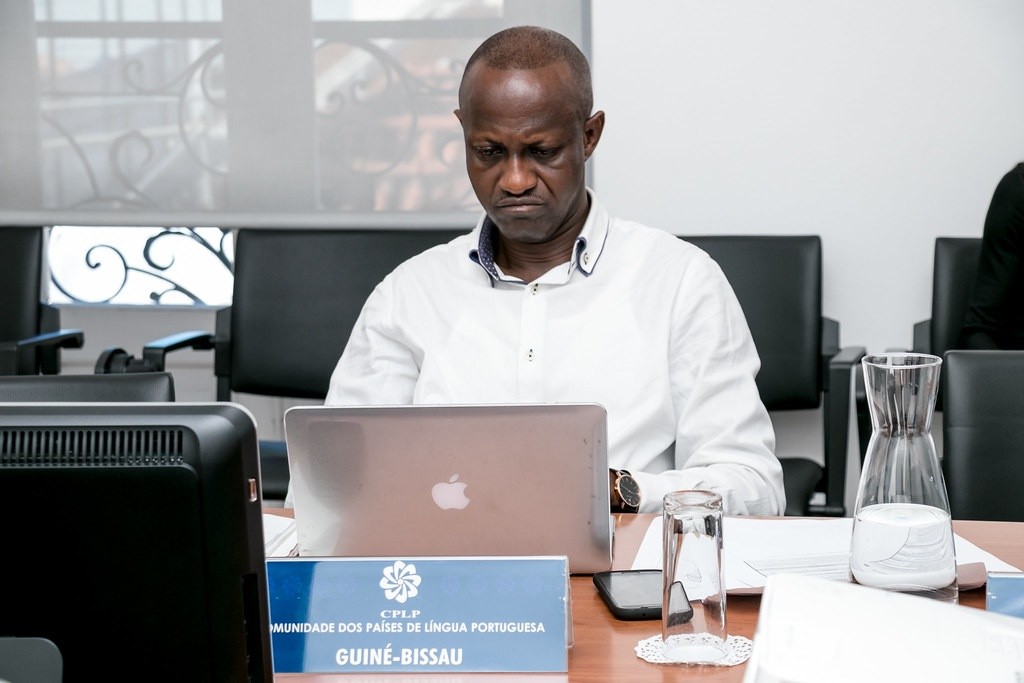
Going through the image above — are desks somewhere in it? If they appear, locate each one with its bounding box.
[262,500,1024,682]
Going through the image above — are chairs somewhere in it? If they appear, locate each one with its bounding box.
[675,233,868,517]
[93,204,473,508]
[906,164,1024,522]
[0,197,85,376]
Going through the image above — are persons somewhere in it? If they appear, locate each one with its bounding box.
[284,27,786,519]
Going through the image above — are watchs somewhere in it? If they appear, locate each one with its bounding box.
[609,466,642,515]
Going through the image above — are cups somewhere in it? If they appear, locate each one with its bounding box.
[661,490,727,646]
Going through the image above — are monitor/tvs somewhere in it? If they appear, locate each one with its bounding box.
[0,400,275,682]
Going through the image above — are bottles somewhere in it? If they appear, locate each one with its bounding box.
[850,350,960,606]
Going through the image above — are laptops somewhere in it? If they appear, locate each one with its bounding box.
[282,401,615,575]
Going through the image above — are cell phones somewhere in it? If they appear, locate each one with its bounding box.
[593,570,692,623]
